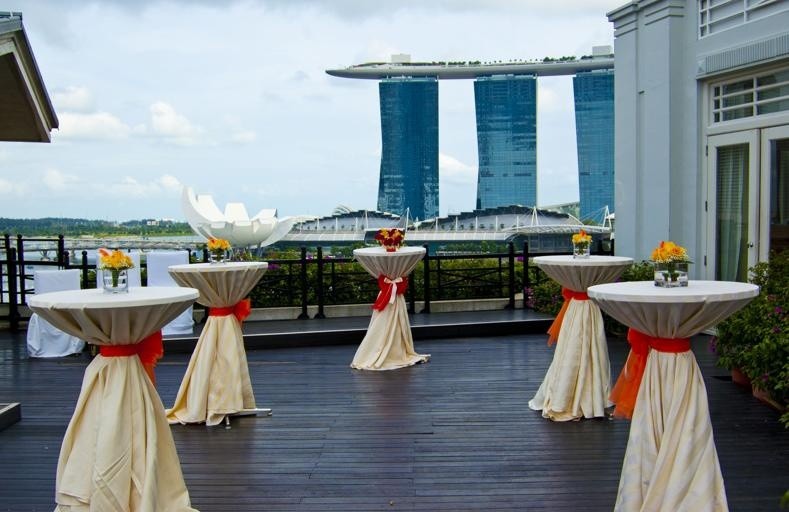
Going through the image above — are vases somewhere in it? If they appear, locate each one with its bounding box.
[653,266,688,287]
[101,267,128,293]
[573,243,590,256]
[208,248,231,262]
[386,243,397,252]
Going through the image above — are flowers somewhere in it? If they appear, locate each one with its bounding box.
[96,246,136,289]
[572,230,593,255]
[374,229,405,252]
[649,241,695,287]
[712,250,789,412]
[206,239,232,262]
